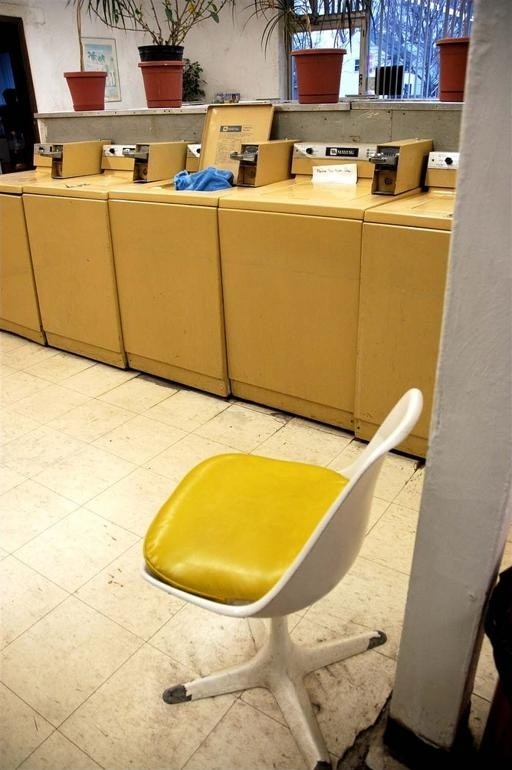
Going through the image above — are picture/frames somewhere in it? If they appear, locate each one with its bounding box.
[80,37,121,102]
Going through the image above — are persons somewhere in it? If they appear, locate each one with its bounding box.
[2,86,19,104]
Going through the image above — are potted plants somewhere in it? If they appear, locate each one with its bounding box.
[88,0,236,108]
[63,0,107,111]
[240,0,376,104]
[435,0,472,102]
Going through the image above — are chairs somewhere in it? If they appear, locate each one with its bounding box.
[142,387,423,769]
